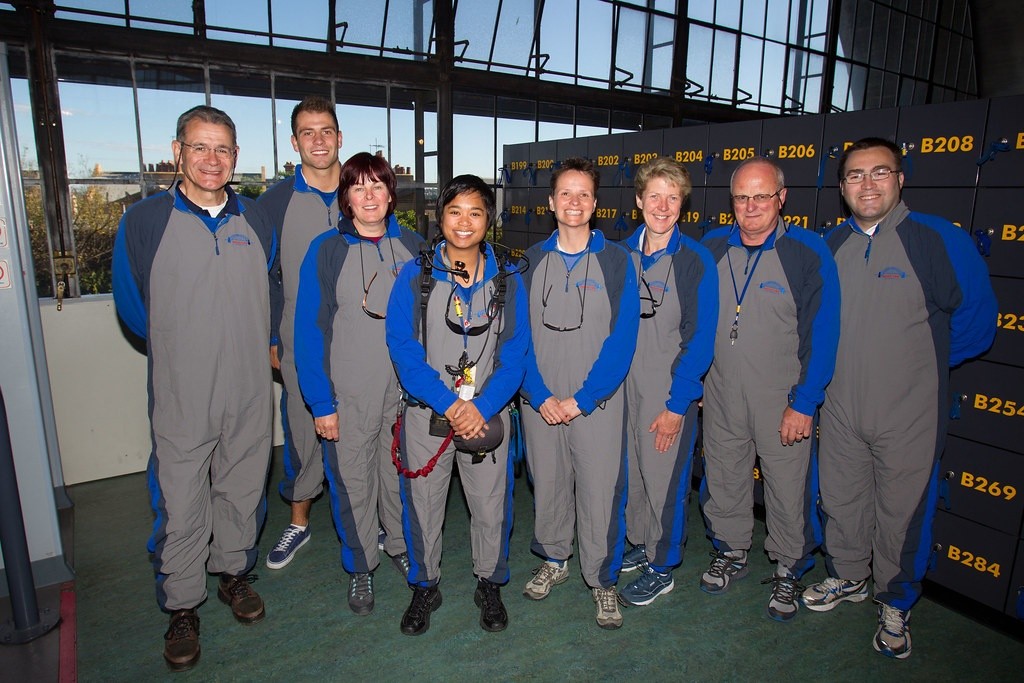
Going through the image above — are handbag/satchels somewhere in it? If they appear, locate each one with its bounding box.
[450,413,505,457]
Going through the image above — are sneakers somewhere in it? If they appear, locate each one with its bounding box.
[347,572,374,615]
[400,587,442,636]
[872,603,911,659]
[393,550,415,589]
[620,544,649,572]
[217,572,264,625]
[619,565,674,607]
[592,586,628,631]
[759,559,808,621]
[162,612,201,671]
[266,524,310,569]
[698,549,749,595]
[521,559,569,601]
[375,521,387,553]
[473,576,508,631]
[801,576,869,611]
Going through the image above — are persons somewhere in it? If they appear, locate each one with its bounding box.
[385,173,532,635]
[515,153,640,630]
[112,105,286,671]
[700,156,843,622]
[256,94,345,568]
[294,152,430,614]
[616,157,719,606]
[802,137,1000,660]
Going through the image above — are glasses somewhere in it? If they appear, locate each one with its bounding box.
[444,287,493,337]
[730,188,783,204]
[840,169,902,184]
[639,275,657,319]
[361,274,387,320]
[180,141,238,159]
[541,284,583,333]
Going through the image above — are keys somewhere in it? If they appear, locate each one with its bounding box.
[730,330,737,346]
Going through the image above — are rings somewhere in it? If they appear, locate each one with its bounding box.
[796,432,803,434]
[667,436,672,439]
[550,419,554,421]
[321,431,325,434]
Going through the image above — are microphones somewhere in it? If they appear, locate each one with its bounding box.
[426,255,468,275]
